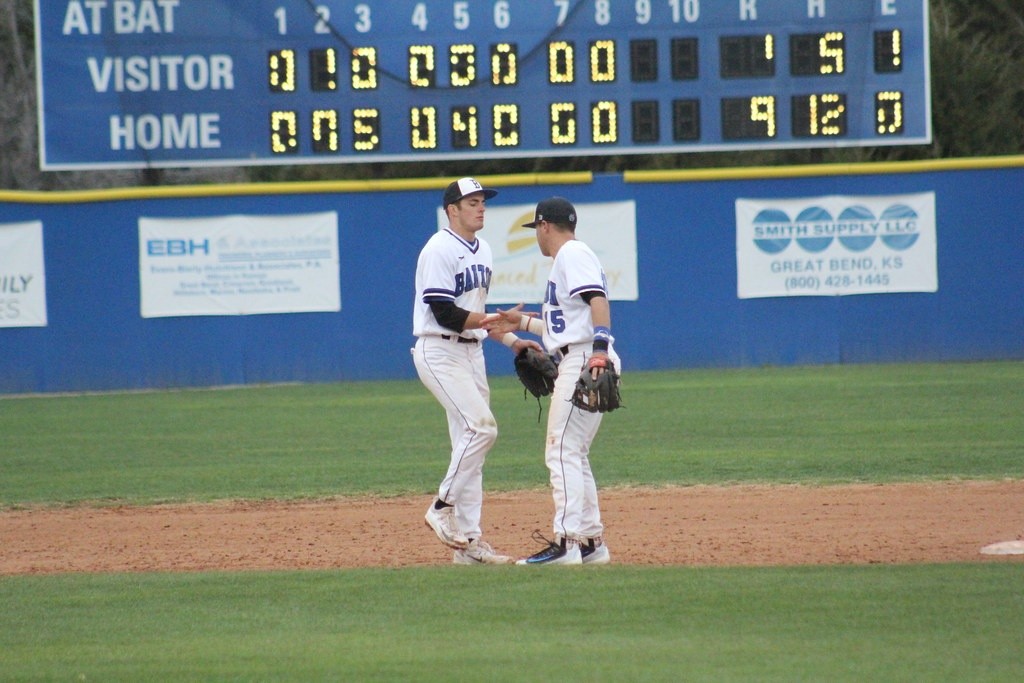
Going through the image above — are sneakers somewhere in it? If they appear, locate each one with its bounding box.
[424,496,468,550]
[515,530,582,568]
[579,537,610,565]
[453,538,514,566]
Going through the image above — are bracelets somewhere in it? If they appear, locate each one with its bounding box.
[501,331,519,349]
[486,313,500,326]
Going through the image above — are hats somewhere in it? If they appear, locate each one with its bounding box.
[521,196,577,228]
[443,177,498,209]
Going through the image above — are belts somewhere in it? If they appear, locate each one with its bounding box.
[549,345,569,368]
[442,334,478,343]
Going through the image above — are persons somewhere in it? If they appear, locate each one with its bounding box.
[479,196,622,566]
[412,178,539,565]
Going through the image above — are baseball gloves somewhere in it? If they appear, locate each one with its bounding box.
[514,347,559,399]
[571,353,620,413]
